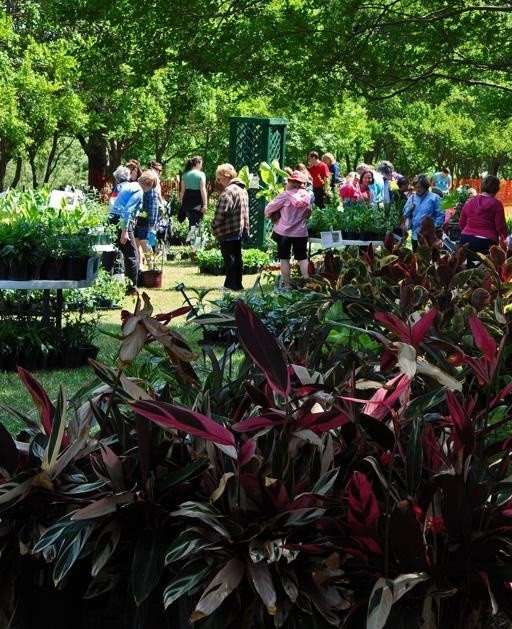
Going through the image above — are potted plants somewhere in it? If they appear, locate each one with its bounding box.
[138,210,279,289]
[306,192,407,242]
[1,176,119,370]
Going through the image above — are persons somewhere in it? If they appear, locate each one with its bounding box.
[148,160,164,204]
[210,163,250,291]
[107,170,157,295]
[109,165,132,204]
[430,167,453,195]
[180,156,208,234]
[283,150,410,211]
[467,188,476,200]
[263,169,312,291]
[402,174,445,251]
[459,175,508,256]
[125,156,157,269]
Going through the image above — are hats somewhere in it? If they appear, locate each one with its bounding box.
[286,169,308,184]
